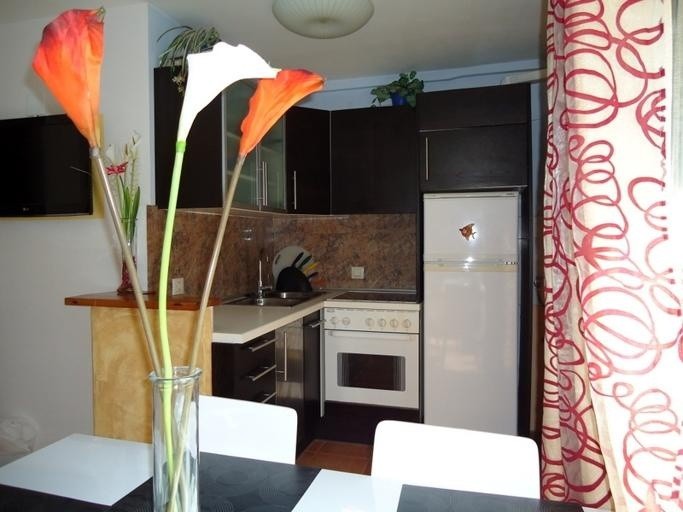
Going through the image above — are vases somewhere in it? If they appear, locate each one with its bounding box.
[118,217,138,295]
[149,364,203,512]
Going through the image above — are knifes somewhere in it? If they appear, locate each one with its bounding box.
[292,252,320,278]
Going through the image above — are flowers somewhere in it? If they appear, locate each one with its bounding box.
[34,5,327,512]
[101,130,142,244]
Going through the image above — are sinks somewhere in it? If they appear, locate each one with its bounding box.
[249,291,328,299]
[223,298,308,307]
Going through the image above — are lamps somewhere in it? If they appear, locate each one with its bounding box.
[272,0,373,39]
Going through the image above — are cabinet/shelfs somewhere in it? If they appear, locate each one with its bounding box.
[211,328,275,406]
[275,311,303,456]
[279,104,328,216]
[414,82,530,189]
[323,104,416,215]
[152,64,288,218]
[303,307,322,453]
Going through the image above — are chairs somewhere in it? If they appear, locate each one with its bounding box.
[197,392,299,464]
[370,418,541,499]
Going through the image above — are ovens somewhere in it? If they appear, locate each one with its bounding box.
[322,329,422,413]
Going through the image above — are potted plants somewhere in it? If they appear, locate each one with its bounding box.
[154,15,219,90]
[371,67,423,105]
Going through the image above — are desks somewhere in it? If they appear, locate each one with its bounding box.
[0,426,605,512]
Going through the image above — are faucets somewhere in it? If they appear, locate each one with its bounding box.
[257,247,273,299]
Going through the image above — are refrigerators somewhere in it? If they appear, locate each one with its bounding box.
[422,190,521,437]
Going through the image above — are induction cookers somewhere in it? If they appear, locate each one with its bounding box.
[320,290,420,335]
